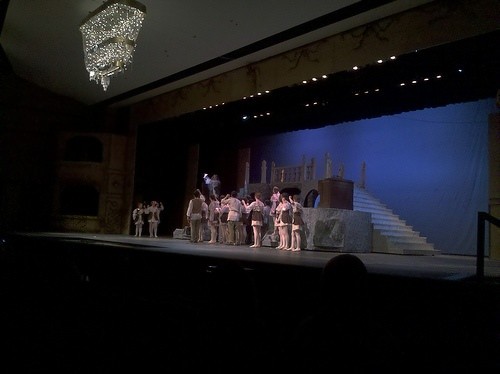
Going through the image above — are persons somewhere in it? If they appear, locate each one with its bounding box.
[199,202,209,242]
[145,201,164,238]
[220,186,304,252]
[208,195,220,244]
[186,189,205,244]
[132,202,145,237]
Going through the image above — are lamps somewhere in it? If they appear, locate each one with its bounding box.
[77,0,146,93]
